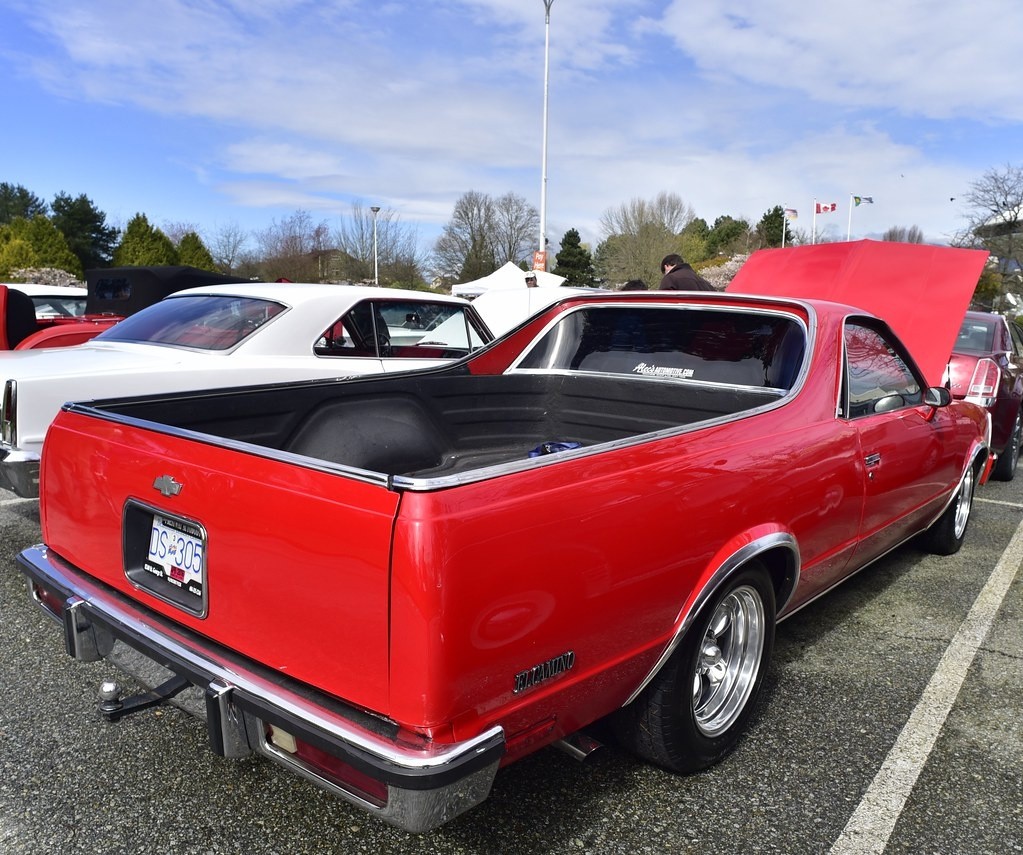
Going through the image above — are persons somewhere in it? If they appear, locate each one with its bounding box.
[619,279,648,290]
[659,254,717,291]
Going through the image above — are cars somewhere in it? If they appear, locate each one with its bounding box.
[936,309,1022,483]
[0,264,437,351]
[1,281,621,499]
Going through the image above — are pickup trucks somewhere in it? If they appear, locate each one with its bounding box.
[13,237,996,838]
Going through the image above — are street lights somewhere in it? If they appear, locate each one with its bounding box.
[540,0,555,272]
[371,206,381,285]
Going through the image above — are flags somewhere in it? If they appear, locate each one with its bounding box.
[784,209,798,219]
[852,195,874,207]
[816,203,836,214]
